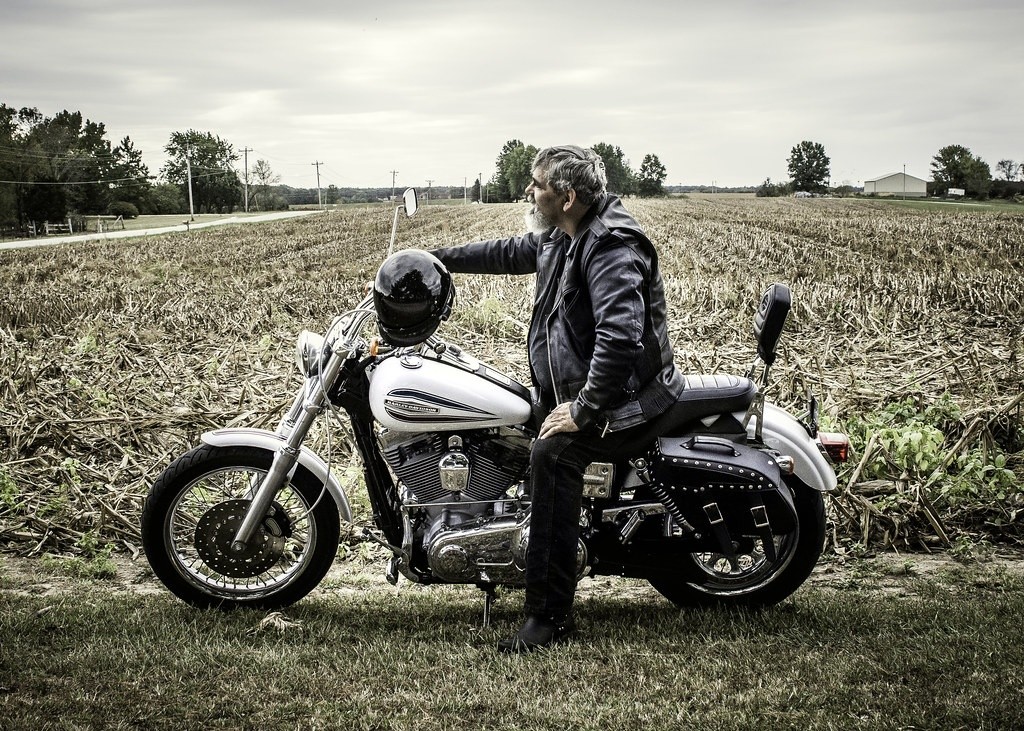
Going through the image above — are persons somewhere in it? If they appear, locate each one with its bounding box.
[422,143,687,652]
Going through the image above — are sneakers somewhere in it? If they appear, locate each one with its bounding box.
[498,614,577,651]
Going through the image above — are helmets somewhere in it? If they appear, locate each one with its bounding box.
[375,248,457,346]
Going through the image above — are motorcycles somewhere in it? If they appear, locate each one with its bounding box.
[140,185,850,619]
